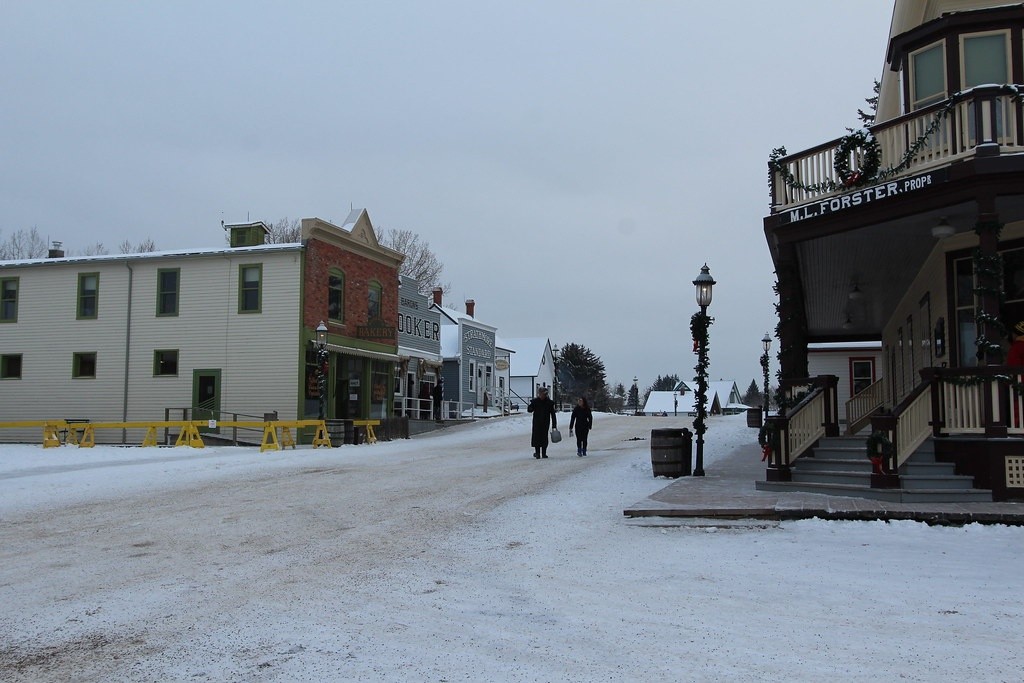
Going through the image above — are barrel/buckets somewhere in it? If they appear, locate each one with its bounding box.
[325,420,345,447]
[650,428,693,479]
[345,419,354,444]
[747,408,761,428]
[354,426,359,445]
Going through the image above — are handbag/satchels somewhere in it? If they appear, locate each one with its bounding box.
[550,426,562,443]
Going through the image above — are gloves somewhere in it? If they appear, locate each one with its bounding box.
[552,424,556,428]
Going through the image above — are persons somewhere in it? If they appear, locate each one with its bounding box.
[568,396,593,456]
[527,386,557,458]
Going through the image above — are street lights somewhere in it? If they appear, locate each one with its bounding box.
[551,343,559,409]
[673,389,678,416]
[632,374,639,416]
[692,261,717,478]
[314,320,329,447]
[760,331,773,421]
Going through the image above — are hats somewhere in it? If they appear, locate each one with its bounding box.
[538,387,549,398]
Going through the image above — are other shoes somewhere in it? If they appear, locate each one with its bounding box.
[577,453,586,456]
[534,453,540,459]
[542,455,548,458]
